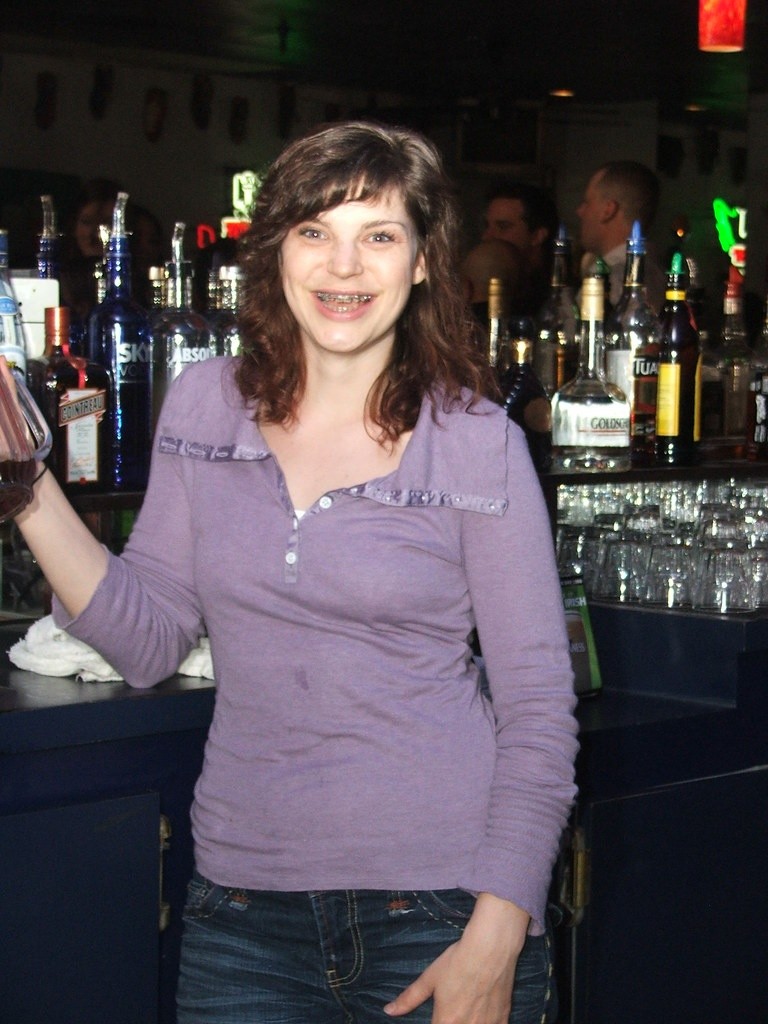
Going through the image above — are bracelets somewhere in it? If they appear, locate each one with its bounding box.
[33,463,49,485]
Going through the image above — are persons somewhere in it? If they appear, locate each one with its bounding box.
[463,239,534,329]
[48,176,141,327]
[575,159,665,309]
[483,182,557,302]
[0,122,580,1024]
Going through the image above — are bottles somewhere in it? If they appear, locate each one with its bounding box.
[700,267,768,462]
[38,196,80,358]
[26,308,111,493]
[143,223,218,462]
[0,230,26,387]
[479,279,552,469]
[80,193,155,493]
[207,238,255,357]
[546,221,630,474]
[616,222,701,470]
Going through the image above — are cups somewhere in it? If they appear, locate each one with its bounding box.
[0,356,53,524]
[556,479,768,614]
